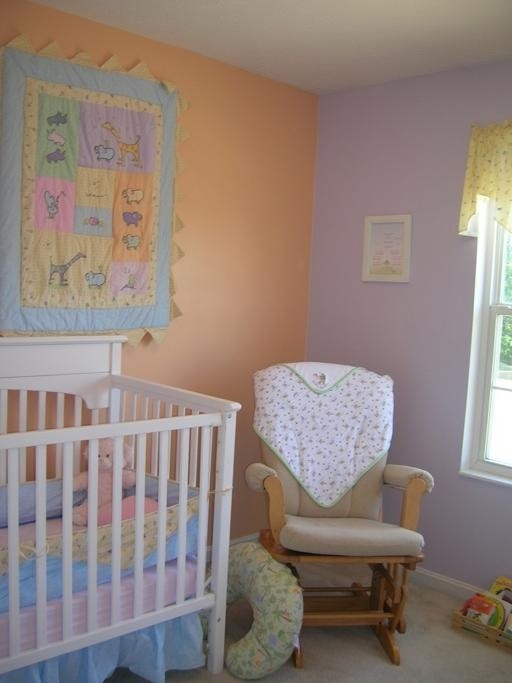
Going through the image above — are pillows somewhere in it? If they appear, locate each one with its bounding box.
[96,495,158,527]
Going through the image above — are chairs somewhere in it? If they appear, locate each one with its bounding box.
[244,362,427,669]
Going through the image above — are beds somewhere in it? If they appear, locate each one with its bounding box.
[1,375,242,682]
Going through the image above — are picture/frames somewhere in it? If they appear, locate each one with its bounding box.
[361,213,413,283]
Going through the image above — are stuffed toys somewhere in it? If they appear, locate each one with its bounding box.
[73,439,136,527]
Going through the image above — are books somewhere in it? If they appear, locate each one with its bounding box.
[461,576,512,645]
